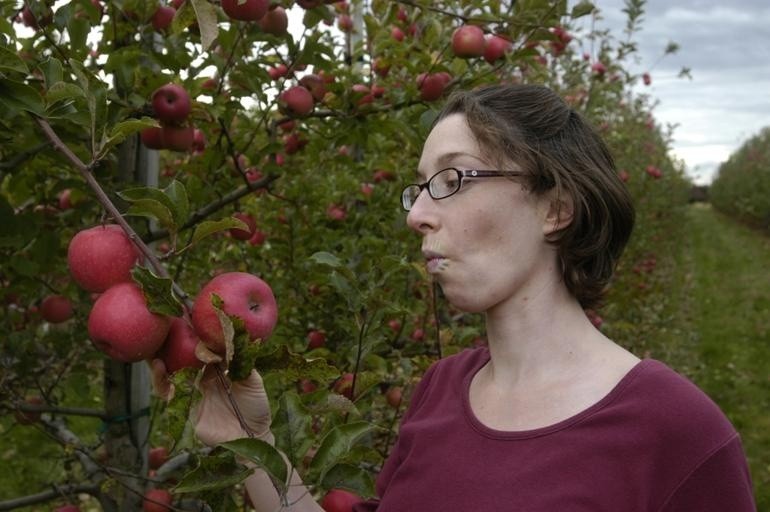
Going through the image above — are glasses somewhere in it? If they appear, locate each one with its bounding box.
[400,168,530,212]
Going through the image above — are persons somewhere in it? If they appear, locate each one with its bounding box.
[148,81,758,511]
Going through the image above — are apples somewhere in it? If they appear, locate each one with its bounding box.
[14,0,667,512]
